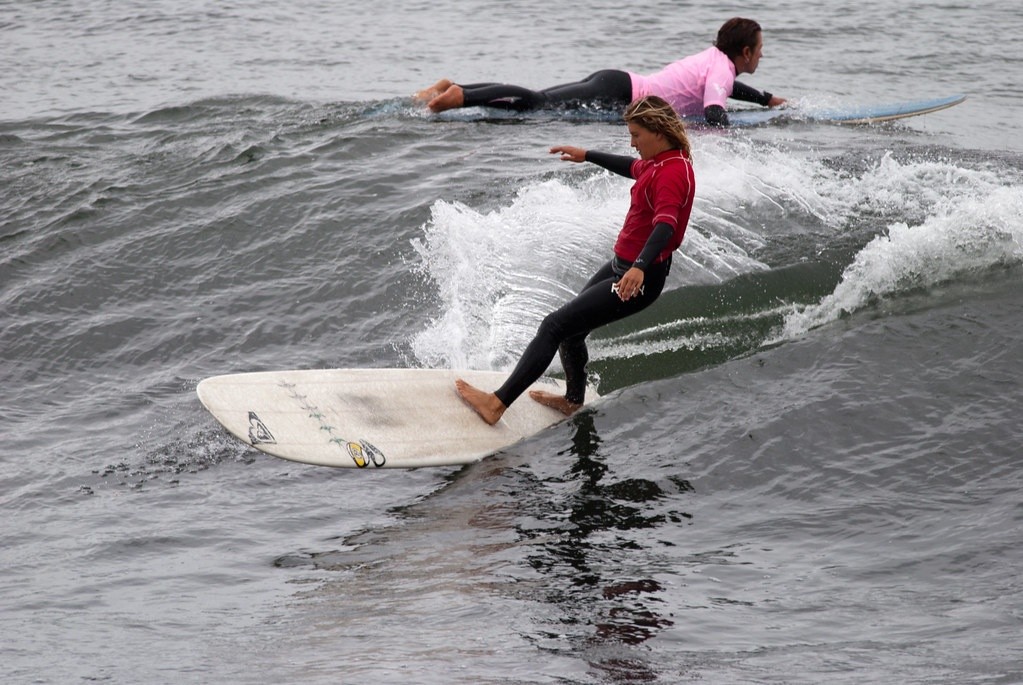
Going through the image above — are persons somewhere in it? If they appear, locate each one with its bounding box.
[424,16,786,125]
[453,96,696,426]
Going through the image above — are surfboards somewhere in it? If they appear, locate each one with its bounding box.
[195,367,601,468]
[436,92,967,131]
